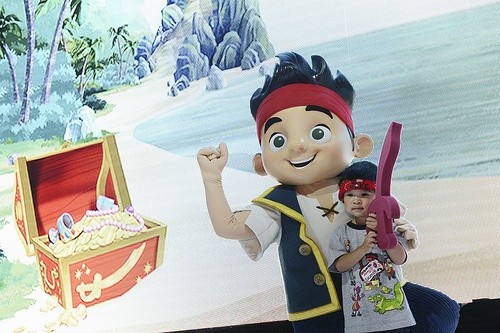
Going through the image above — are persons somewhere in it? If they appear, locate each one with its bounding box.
[197,52,460,333]
[327,161,417,333]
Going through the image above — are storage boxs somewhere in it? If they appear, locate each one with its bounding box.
[10,134,166,310]
